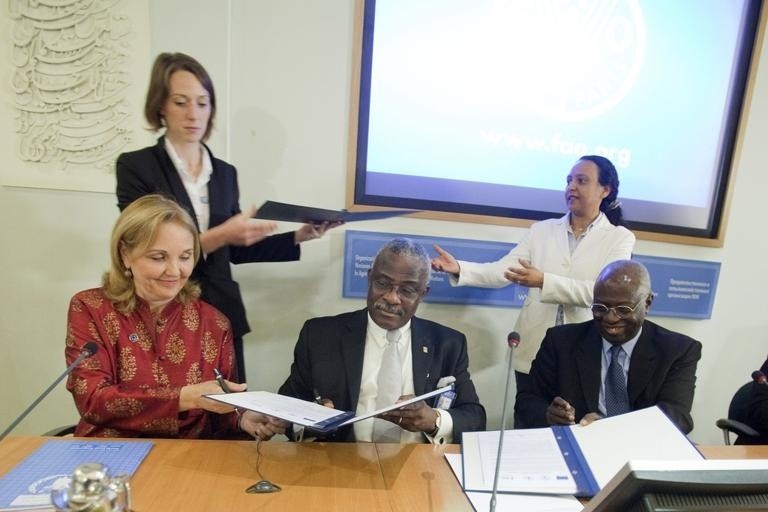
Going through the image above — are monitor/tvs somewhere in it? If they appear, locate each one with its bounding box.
[581,457,768,512]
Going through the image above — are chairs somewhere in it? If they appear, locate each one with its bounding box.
[40,425,77,436]
[716,418,761,446]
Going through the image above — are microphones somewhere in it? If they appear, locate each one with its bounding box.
[489,332,520,512]
[0,341,97,442]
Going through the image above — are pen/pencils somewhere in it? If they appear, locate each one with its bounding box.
[212,368,232,393]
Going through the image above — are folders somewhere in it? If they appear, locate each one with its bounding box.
[252,200,423,224]
[201,383,455,435]
[461,404,707,498]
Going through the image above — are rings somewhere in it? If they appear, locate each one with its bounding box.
[398,417,402,424]
[518,280,520,284]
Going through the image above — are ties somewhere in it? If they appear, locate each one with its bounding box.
[372,329,402,442]
[605,346,630,417]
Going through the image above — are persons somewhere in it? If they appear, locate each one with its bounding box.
[514,259,702,435]
[116,52,346,391]
[64,193,288,441]
[278,237,487,444]
[432,155,636,429]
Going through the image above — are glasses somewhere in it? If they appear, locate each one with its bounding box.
[372,280,418,299]
[590,296,648,319]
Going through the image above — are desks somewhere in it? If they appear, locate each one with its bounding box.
[1,432,768,511]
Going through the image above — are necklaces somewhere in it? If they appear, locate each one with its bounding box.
[571,223,589,231]
